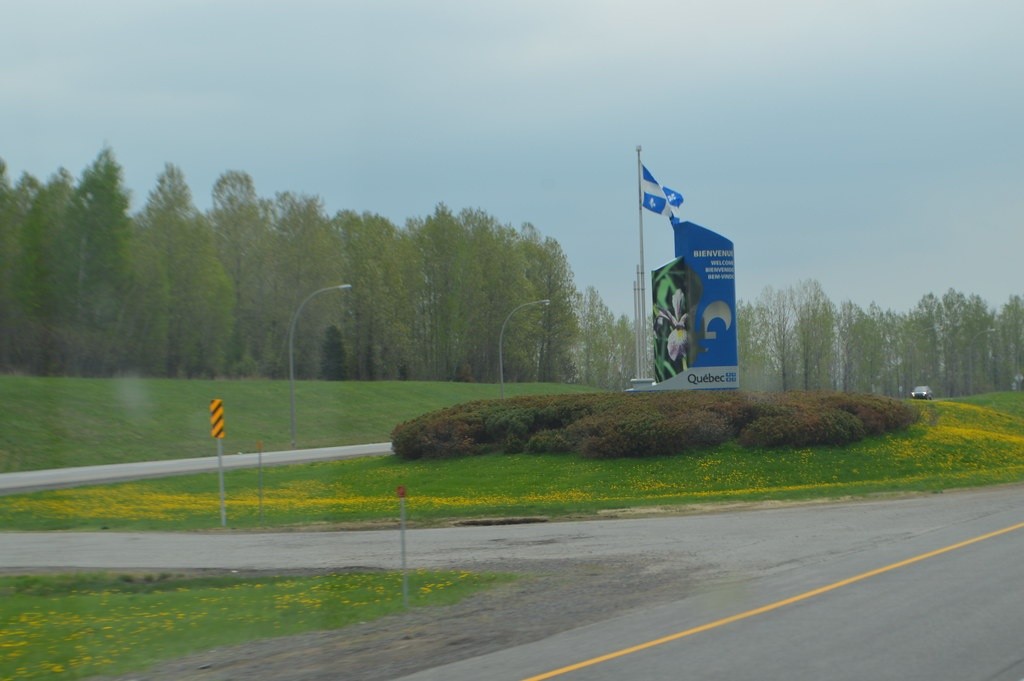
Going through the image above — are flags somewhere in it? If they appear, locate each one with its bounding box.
[638,160,683,231]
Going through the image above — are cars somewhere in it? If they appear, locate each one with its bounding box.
[911,385,933,400]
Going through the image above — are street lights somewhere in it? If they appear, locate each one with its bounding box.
[290,284,351,449]
[499,299,551,401]
